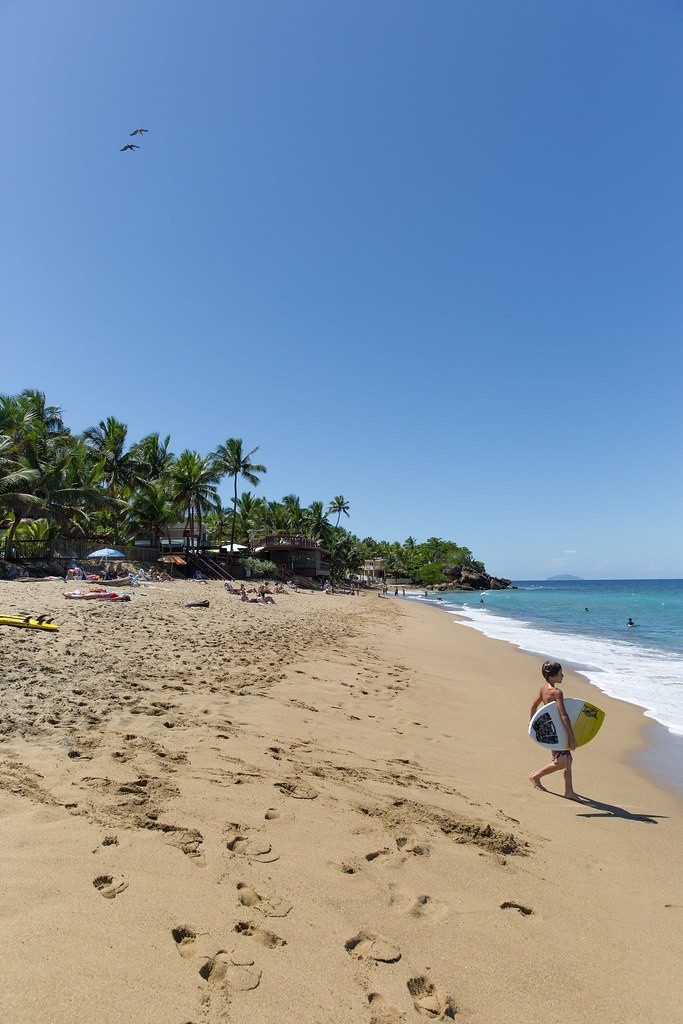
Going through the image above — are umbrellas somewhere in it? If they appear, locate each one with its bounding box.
[88,548,125,562]
[162,556,187,575]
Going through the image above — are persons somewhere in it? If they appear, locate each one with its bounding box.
[110,562,128,579]
[224,581,284,605]
[529,661,584,803]
[62,592,130,601]
[10,565,21,582]
[324,577,354,595]
[383,586,388,594]
[395,587,398,596]
[71,559,77,569]
[149,566,172,582]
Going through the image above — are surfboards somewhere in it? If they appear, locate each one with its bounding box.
[0,613,61,631]
[527,696,606,751]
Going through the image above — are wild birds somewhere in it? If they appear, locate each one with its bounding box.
[130,128,148,137]
[120,143,141,153]
